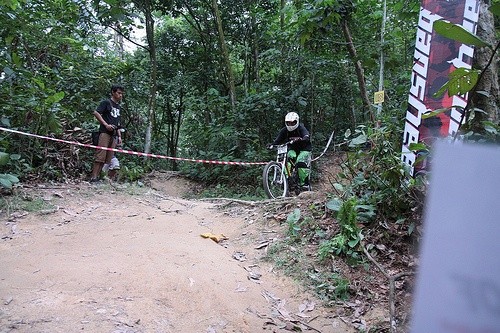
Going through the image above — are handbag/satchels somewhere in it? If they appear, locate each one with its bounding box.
[93,131,99,146]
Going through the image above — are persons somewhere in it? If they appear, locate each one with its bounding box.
[89,84,124,181]
[266,112,312,189]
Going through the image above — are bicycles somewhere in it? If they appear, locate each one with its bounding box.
[263,138,313,199]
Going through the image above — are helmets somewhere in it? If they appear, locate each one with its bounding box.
[285,112,299,132]
